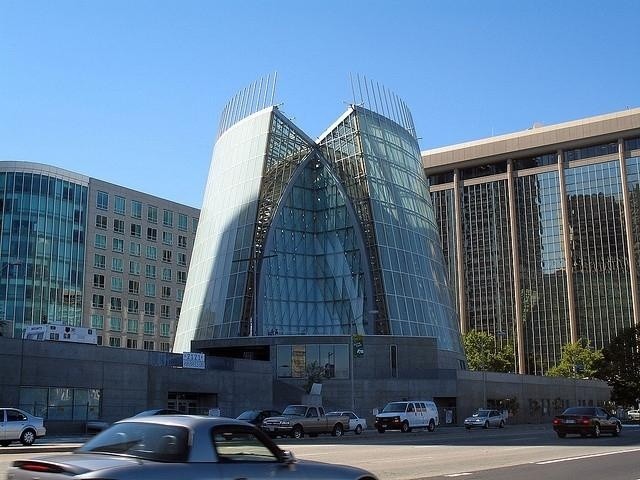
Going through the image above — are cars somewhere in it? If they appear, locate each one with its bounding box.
[129,403,370,440]
[6,412,380,479]
[552,405,624,438]
[0,407,47,446]
[626,409,640,421]
[464,408,508,430]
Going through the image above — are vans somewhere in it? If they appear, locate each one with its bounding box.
[374,400,440,434]
